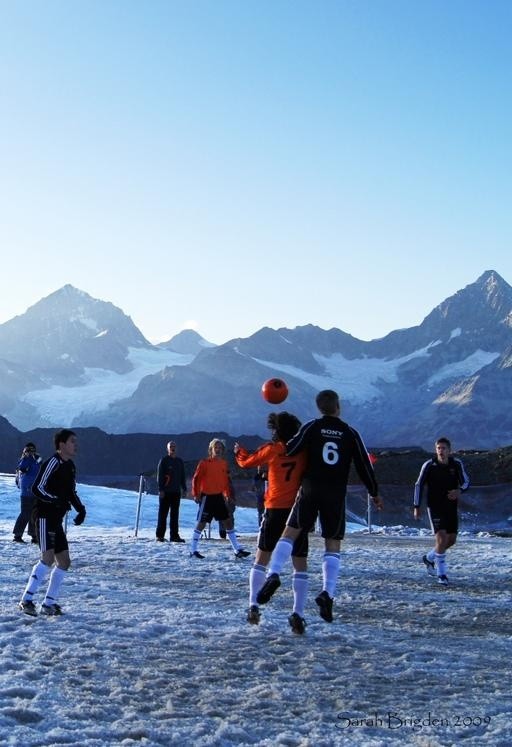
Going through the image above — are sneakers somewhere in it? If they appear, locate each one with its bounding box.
[437,575,449,587]
[39,602,64,616]
[170,537,185,543]
[247,605,262,626]
[17,599,39,618]
[314,591,335,624]
[234,550,252,559]
[256,573,282,605]
[12,536,26,544]
[30,538,40,544]
[423,555,437,577]
[155,538,168,543]
[190,551,206,560]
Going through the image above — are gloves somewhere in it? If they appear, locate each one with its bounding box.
[72,510,86,526]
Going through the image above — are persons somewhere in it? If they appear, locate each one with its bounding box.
[155,439,187,543]
[411,436,472,586]
[218,461,318,537]
[16,429,87,617]
[255,389,384,622]
[12,447,40,546]
[231,411,310,635]
[24,443,43,464]
[189,439,252,559]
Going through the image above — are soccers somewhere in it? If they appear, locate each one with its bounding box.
[262,378,288,404]
